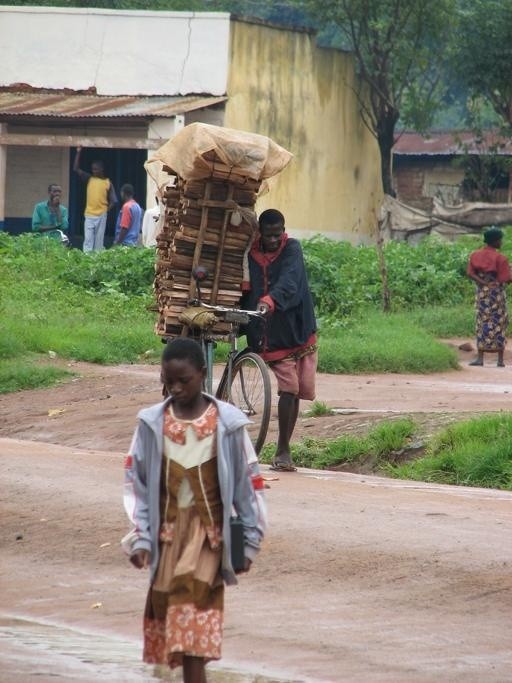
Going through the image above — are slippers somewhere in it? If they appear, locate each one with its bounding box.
[268,453,300,473]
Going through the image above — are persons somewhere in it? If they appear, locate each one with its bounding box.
[121,341,267,683]
[34,184,70,249]
[467,229,512,367]
[238,209,318,471]
[113,184,142,248]
[72,143,117,254]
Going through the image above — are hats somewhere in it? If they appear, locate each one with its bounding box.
[483,228,504,243]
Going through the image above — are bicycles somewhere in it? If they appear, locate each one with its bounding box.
[171,266,273,456]
